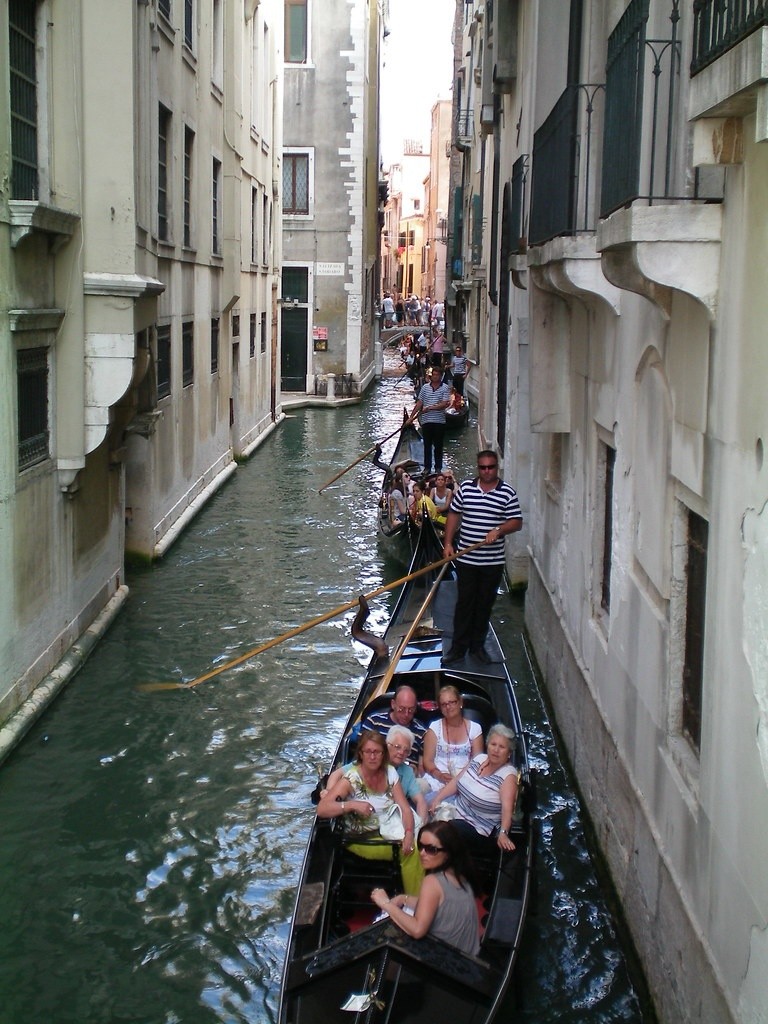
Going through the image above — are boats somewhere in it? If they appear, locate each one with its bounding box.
[277,499,533,1024]
[400,344,470,424]
[376,407,461,566]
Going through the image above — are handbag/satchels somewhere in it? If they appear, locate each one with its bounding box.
[379,803,423,841]
[430,802,455,823]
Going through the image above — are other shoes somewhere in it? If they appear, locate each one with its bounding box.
[436,467,442,473]
[470,647,491,665]
[441,649,465,664]
[422,467,431,474]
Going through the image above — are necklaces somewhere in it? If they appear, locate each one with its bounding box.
[444,718,473,775]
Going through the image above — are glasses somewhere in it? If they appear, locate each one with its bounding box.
[361,748,385,757]
[439,699,460,707]
[393,704,416,714]
[403,474,410,479]
[445,476,452,479]
[389,743,412,756]
[417,842,447,856]
[478,464,496,470]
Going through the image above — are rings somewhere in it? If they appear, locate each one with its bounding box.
[374,887,379,890]
[371,892,374,895]
[410,846,414,851]
[369,807,374,811]
[492,539,495,541]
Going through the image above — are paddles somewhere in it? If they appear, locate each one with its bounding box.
[189,541,487,685]
[396,335,441,387]
[320,411,424,491]
[347,554,451,736]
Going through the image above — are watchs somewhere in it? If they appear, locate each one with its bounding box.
[495,526,504,534]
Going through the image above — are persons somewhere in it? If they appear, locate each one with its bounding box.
[350,685,427,778]
[388,464,456,534]
[423,686,483,792]
[372,820,481,960]
[382,292,446,327]
[440,450,523,664]
[445,346,472,396]
[406,366,451,474]
[431,724,518,849]
[392,283,398,295]
[321,725,429,829]
[317,730,426,898]
[398,329,447,369]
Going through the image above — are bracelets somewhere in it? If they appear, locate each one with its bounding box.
[341,801,345,811]
[499,829,508,834]
[404,895,408,904]
[379,898,391,909]
